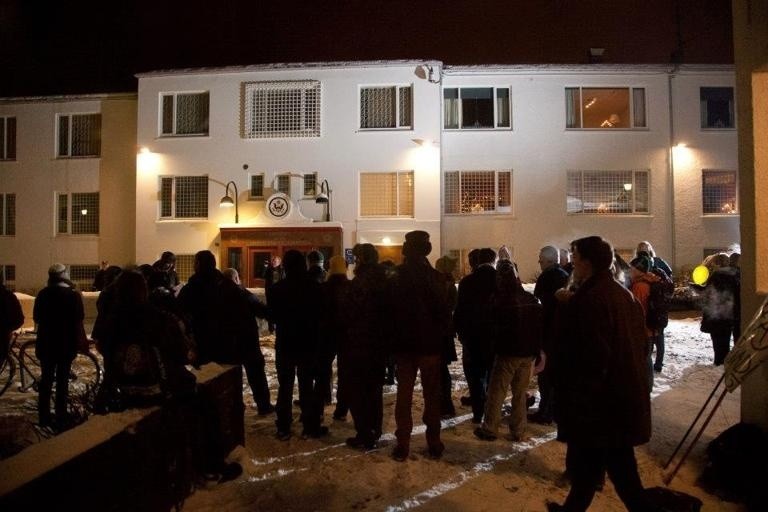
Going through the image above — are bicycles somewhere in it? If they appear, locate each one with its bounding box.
[1,328,100,406]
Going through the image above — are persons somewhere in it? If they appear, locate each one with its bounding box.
[1,229,740,510]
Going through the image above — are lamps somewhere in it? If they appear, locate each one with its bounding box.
[428,66,442,84]
[219,180,239,223]
[314,177,330,222]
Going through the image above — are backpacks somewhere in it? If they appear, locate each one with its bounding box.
[647,277,670,329]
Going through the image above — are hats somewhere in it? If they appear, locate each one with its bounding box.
[402,230,434,257]
[47,262,66,280]
[631,256,650,275]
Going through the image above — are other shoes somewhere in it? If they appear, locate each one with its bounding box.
[208,462,243,486]
[259,397,550,460]
[546,502,564,512]
